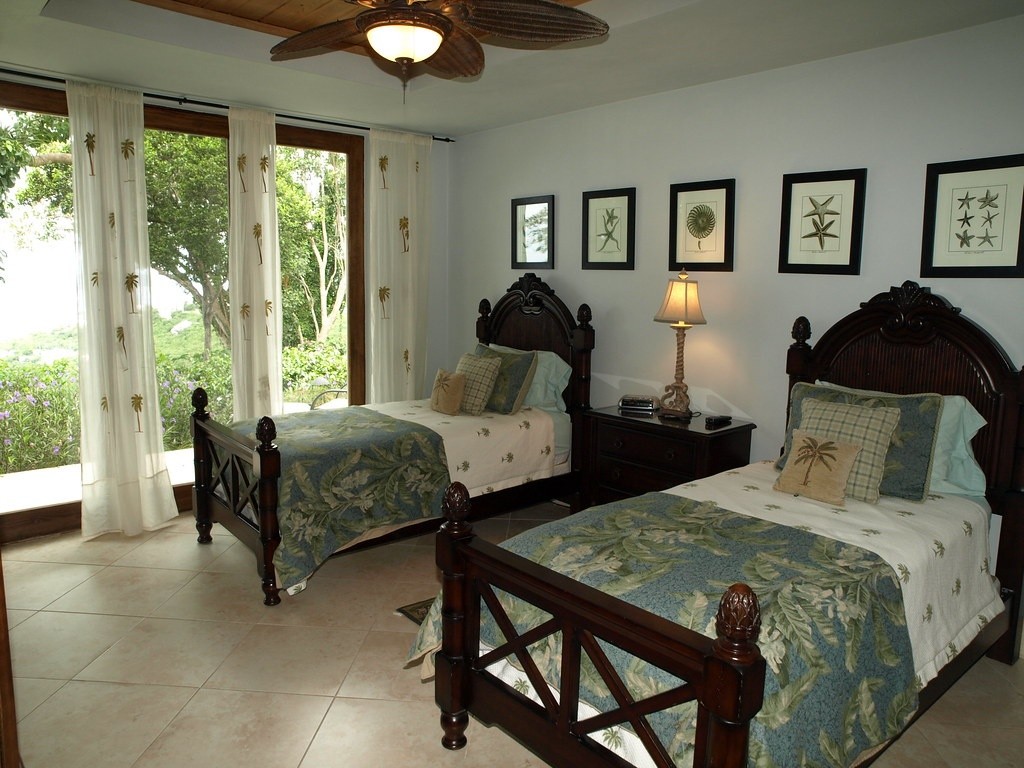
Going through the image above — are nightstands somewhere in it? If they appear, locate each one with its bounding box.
[584,405,756,506]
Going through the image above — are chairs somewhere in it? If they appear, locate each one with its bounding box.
[310,382,347,409]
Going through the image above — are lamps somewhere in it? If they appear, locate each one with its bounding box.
[352,6,454,105]
[653,268,707,418]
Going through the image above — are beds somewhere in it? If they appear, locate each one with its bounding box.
[188,272,595,606]
[407,278,1024,768]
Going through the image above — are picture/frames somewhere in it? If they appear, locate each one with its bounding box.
[510,195,555,270]
[668,179,735,273]
[582,187,635,270]
[919,152,1024,280]
[778,168,867,275]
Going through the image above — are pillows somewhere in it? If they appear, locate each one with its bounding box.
[428,367,467,416]
[814,380,987,497]
[487,344,573,413]
[455,352,502,417]
[773,381,943,505]
[772,428,863,507]
[800,397,900,505]
[474,343,538,415]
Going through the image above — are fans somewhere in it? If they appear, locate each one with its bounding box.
[269,0,610,77]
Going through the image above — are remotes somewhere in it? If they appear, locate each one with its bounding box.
[705,415,732,424]
